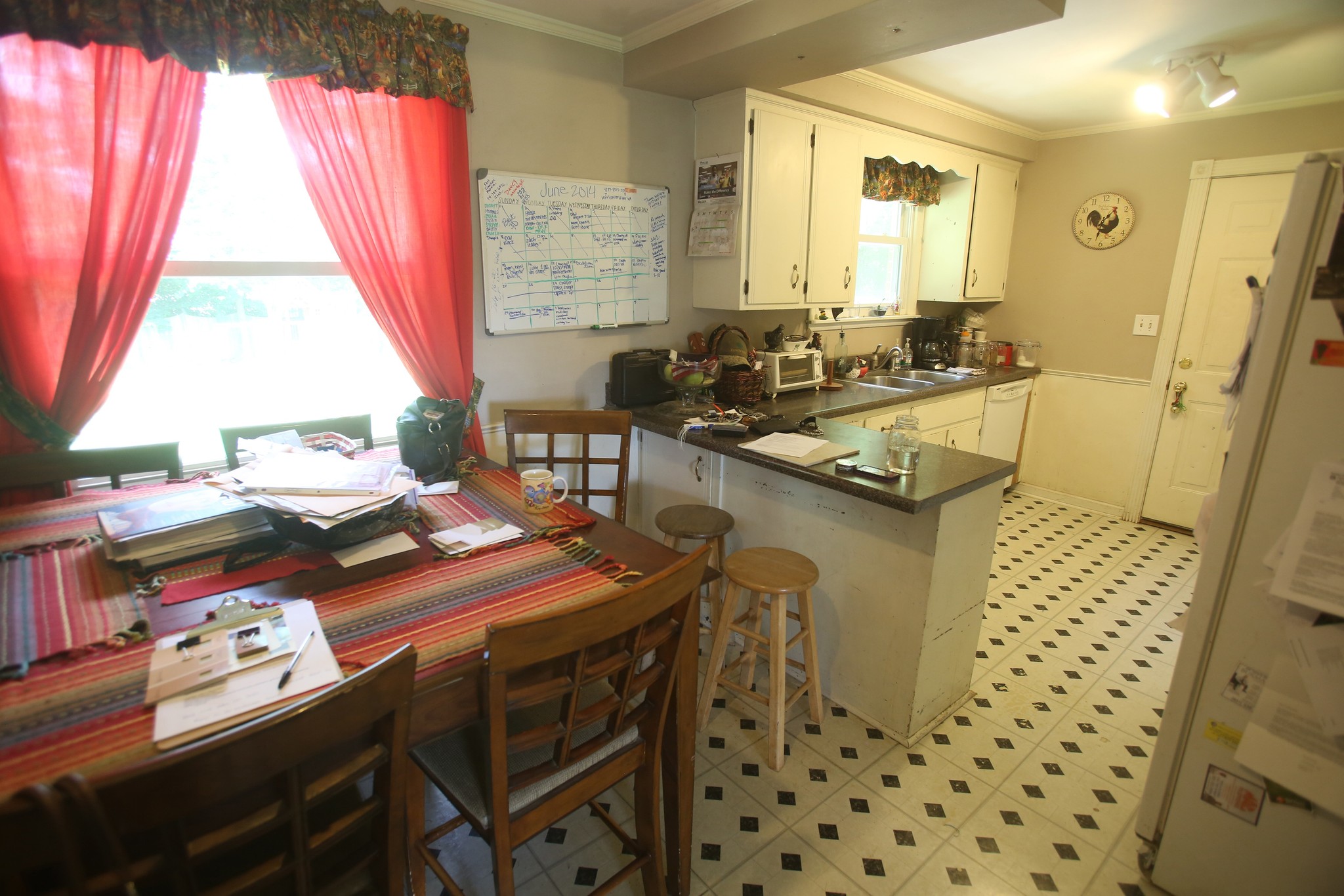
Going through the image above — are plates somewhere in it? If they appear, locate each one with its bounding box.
[971,339,991,342]
[957,326,973,333]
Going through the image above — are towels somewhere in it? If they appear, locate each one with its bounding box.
[947,364,987,375]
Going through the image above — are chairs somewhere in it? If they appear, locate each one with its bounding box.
[0,408,710,896]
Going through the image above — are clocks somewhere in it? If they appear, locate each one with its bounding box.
[1073,193,1135,250]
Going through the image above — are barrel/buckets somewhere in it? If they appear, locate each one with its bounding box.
[998,341,1013,366]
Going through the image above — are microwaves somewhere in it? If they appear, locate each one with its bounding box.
[756,348,823,399]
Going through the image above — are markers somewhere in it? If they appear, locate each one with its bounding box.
[592,324,619,329]
[683,424,714,429]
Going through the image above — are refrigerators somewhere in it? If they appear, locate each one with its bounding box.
[1133,150,1344,896]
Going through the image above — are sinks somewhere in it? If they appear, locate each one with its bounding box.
[852,375,934,390]
[886,370,966,383]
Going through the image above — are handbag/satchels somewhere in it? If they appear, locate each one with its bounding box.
[396,396,466,486]
[751,414,824,436]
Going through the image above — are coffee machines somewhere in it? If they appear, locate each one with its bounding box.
[910,316,952,371]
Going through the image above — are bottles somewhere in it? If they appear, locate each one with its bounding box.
[997,343,1007,368]
[989,342,998,366]
[956,342,972,368]
[887,415,922,474]
[1015,340,1042,368]
[971,343,991,369]
[959,329,971,360]
[833,332,848,379]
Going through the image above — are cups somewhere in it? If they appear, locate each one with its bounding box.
[974,331,987,341]
[521,469,568,514]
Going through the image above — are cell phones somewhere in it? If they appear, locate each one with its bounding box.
[853,465,900,482]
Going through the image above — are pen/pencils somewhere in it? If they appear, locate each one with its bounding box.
[278,629,313,688]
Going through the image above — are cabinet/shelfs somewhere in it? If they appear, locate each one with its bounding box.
[637,434,714,624]
[920,163,1019,302]
[695,90,811,310]
[866,392,984,455]
[804,110,860,307]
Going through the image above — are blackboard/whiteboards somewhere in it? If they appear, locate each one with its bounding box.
[477,168,670,337]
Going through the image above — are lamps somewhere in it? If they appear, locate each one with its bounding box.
[1156,61,1197,115]
[1197,53,1240,110]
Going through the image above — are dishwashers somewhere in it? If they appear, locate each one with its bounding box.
[977,378,1033,488]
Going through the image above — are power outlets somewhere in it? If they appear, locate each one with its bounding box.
[1133,315,1160,337]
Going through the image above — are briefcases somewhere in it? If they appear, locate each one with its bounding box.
[611,349,676,407]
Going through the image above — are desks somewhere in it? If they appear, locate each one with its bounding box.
[0,435,703,896]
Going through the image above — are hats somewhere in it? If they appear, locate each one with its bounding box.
[716,329,753,370]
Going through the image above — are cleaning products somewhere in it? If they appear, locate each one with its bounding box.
[901,337,914,369]
[890,338,902,371]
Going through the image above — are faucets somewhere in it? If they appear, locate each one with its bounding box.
[869,343,904,368]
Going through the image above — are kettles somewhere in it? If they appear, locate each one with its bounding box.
[921,338,951,364]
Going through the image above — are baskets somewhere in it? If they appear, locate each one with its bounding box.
[711,325,765,402]
[299,431,357,460]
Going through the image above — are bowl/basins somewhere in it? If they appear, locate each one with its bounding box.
[781,335,810,351]
[872,306,888,317]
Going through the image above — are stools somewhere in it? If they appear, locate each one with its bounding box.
[696,547,824,773]
[654,505,733,711]
